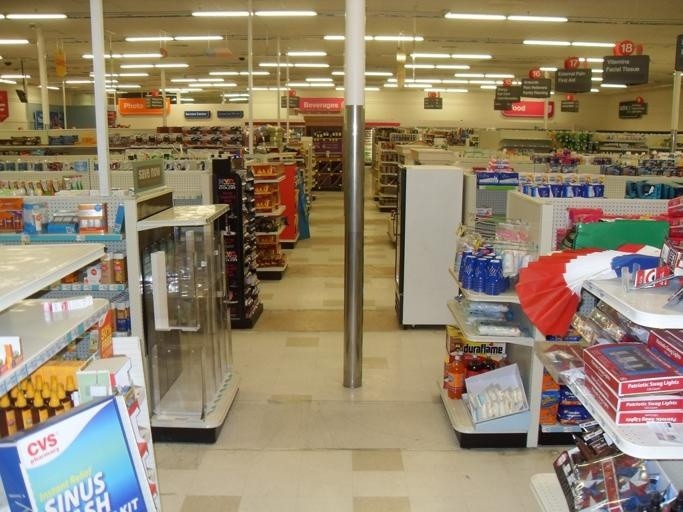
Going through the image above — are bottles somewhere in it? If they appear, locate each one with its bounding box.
[0,173,84,199]
[99,250,131,333]
[4,342,15,370]
[448,343,508,401]
[0,370,82,441]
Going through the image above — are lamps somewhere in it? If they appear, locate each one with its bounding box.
[0,11,628,101]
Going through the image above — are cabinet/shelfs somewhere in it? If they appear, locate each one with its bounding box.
[372,125,682,508]
[307,117,349,191]
[0,118,318,508]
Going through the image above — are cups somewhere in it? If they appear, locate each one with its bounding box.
[458,245,509,296]
